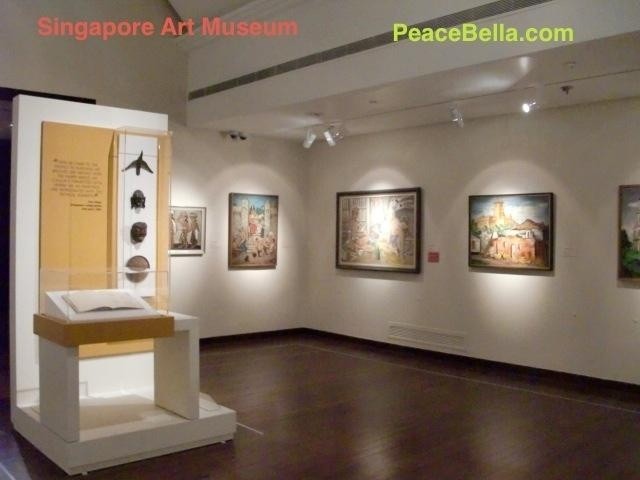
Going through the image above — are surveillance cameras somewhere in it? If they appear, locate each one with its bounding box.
[240,133,247,140]
[228,132,238,139]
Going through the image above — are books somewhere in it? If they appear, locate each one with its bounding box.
[61,288,151,314]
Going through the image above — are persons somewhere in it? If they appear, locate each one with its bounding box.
[233,226,276,260]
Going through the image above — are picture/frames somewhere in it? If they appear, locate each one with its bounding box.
[168,191,280,271]
[333,185,422,275]
[616,183,640,289]
[467,191,556,275]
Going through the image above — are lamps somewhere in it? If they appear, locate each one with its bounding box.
[301,122,341,154]
